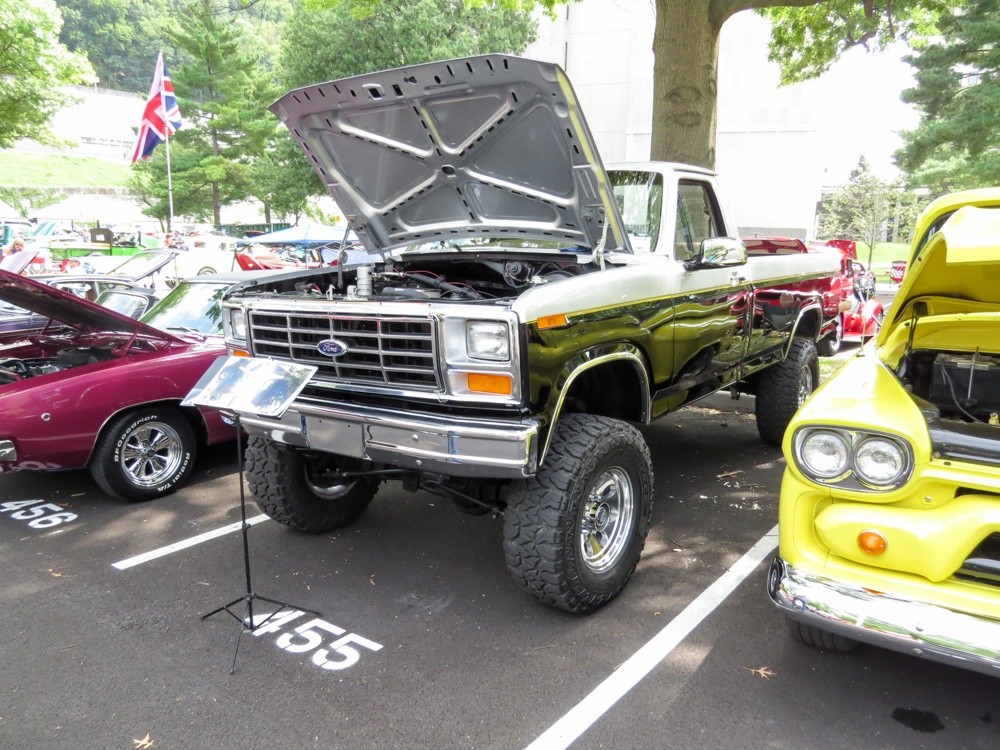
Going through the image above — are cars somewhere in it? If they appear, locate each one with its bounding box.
[0,216,294,336]
[0,266,309,504]
[765,185,1000,680]
[741,237,884,358]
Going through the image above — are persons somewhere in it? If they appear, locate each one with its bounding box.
[164,230,196,249]
[8,238,24,256]
[56,227,91,242]
[57,257,87,293]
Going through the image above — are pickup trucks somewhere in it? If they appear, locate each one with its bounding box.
[180,53,848,616]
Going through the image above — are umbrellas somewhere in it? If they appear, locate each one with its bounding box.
[28,196,166,228]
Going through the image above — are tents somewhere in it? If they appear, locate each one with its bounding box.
[231,221,400,273]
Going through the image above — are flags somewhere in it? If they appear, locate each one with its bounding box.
[132,49,182,167]
